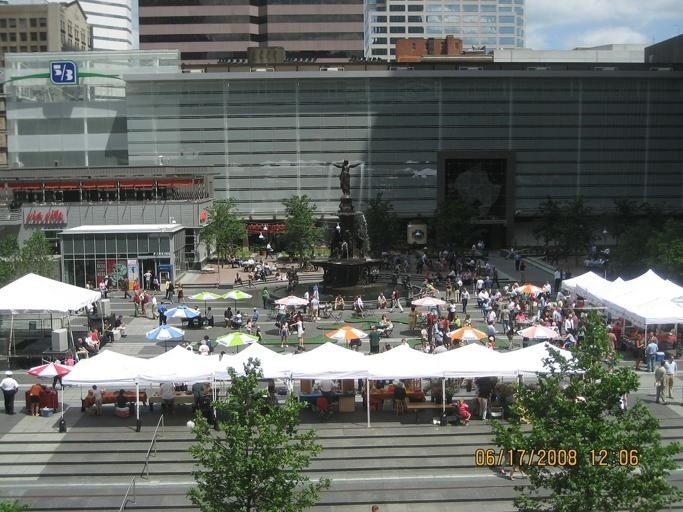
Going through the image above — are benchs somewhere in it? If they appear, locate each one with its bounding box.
[5,351,45,367]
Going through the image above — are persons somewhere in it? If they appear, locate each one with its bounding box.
[591,243,610,269]
[605,317,679,403]
[50,373,64,389]
[332,159,362,194]
[70,240,588,418]
[29,383,44,416]
[0,370,18,414]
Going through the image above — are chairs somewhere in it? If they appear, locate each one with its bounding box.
[180,315,215,329]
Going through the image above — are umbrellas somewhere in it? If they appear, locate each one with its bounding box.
[26,362,71,378]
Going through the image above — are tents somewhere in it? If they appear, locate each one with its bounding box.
[557,269,683,357]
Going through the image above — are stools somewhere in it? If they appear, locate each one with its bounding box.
[114,406,130,417]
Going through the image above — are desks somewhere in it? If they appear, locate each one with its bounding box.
[148,387,460,425]
[622,333,678,364]
[25,386,58,413]
[83,390,147,407]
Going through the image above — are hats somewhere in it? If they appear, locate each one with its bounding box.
[5,371,13,376]
